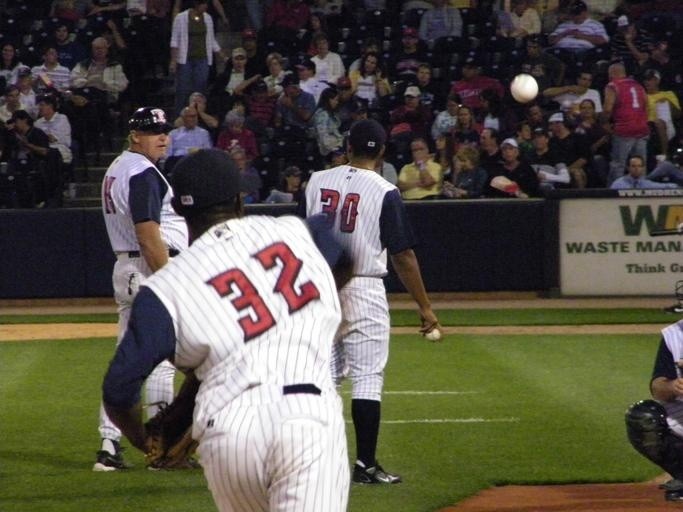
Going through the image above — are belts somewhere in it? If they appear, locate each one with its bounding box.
[283,383,322,394]
[128,248,179,257]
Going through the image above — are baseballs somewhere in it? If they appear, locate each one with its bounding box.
[426,329,441,343]
[511,74,537,102]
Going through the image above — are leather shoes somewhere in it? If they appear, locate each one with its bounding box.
[663,489,682,501]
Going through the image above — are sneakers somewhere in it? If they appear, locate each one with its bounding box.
[352,459,400,484]
[91,450,133,471]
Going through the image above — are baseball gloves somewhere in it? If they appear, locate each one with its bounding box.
[145,370,199,468]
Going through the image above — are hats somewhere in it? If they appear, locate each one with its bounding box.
[615,15,632,31]
[276,72,300,86]
[337,77,351,89]
[400,27,419,38]
[403,86,421,97]
[643,69,660,79]
[170,148,241,216]
[348,118,386,147]
[499,138,518,148]
[17,67,30,77]
[6,110,28,125]
[231,48,246,58]
[284,166,302,177]
[295,60,316,75]
[548,112,565,122]
[241,29,256,39]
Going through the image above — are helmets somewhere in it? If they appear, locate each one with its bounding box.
[128,106,177,131]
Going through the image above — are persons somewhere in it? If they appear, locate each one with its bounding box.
[102,147,350,511]
[625,302,683,502]
[304,119,445,486]
[0,2,682,201]
[95,106,202,474]
